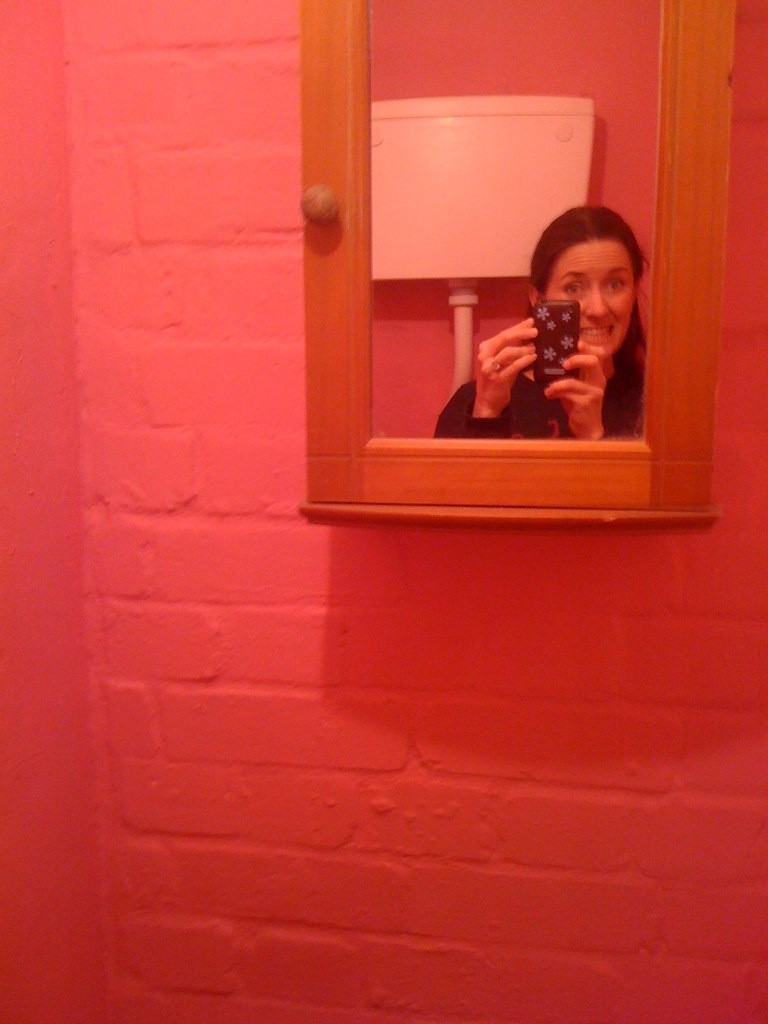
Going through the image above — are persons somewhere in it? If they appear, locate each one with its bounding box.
[433,205,643,441]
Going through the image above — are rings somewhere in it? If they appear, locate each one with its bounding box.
[491,359,501,371]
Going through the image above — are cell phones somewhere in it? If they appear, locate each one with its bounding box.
[530,299,581,383]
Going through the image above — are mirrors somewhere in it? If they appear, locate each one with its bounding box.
[301,0,735,522]
